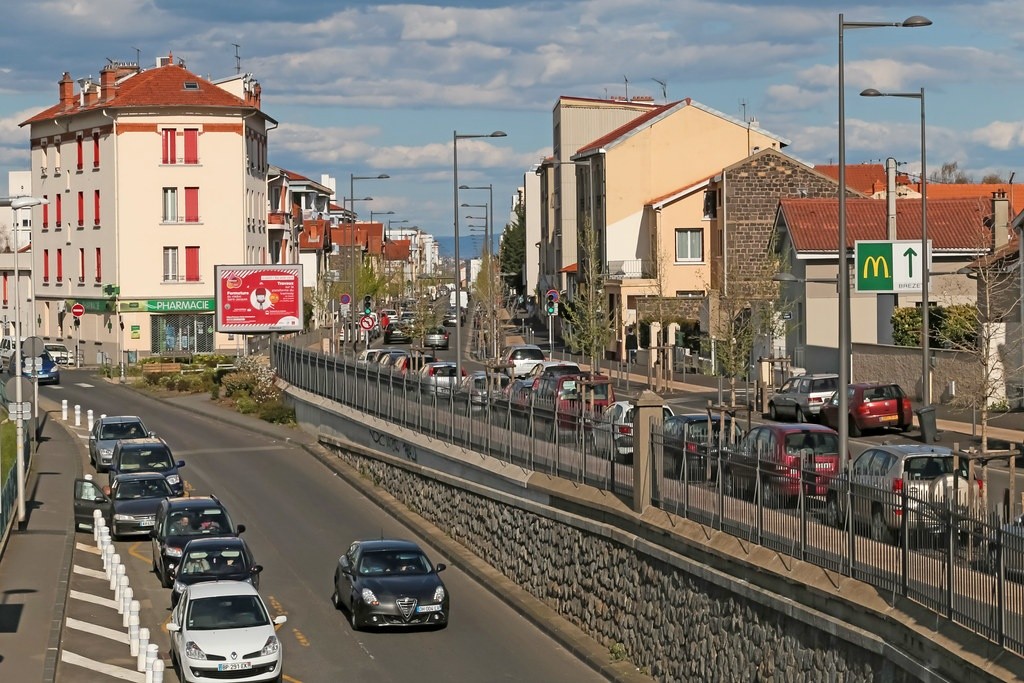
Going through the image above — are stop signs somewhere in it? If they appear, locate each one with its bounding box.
[71,303,85,318]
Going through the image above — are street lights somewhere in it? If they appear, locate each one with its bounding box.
[24,196,50,442]
[340,195,442,333]
[452,129,506,385]
[542,156,596,378]
[350,173,391,349]
[837,13,935,526]
[9,194,42,534]
[858,85,932,407]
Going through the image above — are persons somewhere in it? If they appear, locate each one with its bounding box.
[170,516,194,533]
[198,516,223,531]
[126,425,140,435]
[184,558,210,573]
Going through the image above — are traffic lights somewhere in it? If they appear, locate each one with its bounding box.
[364,295,371,350]
[547,294,554,362]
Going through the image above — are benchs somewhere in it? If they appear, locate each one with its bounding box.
[142,363,181,377]
[177,363,207,375]
[212,364,238,372]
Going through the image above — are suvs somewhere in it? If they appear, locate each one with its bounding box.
[152,493,246,589]
[108,437,185,501]
[767,371,839,424]
[521,371,616,442]
[825,443,988,546]
[495,344,546,382]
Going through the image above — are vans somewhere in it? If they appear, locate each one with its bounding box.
[0,336,29,364]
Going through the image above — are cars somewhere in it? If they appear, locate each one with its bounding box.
[662,413,743,479]
[723,424,856,511]
[336,282,525,338]
[422,324,451,350]
[88,414,157,474]
[338,322,366,347]
[165,536,264,613]
[0,356,4,373]
[391,354,439,381]
[589,401,676,463]
[453,371,511,414]
[357,349,383,365]
[384,321,413,344]
[332,539,450,633]
[486,379,533,436]
[72,472,177,542]
[524,361,582,381]
[7,348,59,386]
[980,510,1024,583]
[164,580,288,683]
[372,348,407,368]
[43,343,75,366]
[417,361,469,400]
[819,381,914,438]
